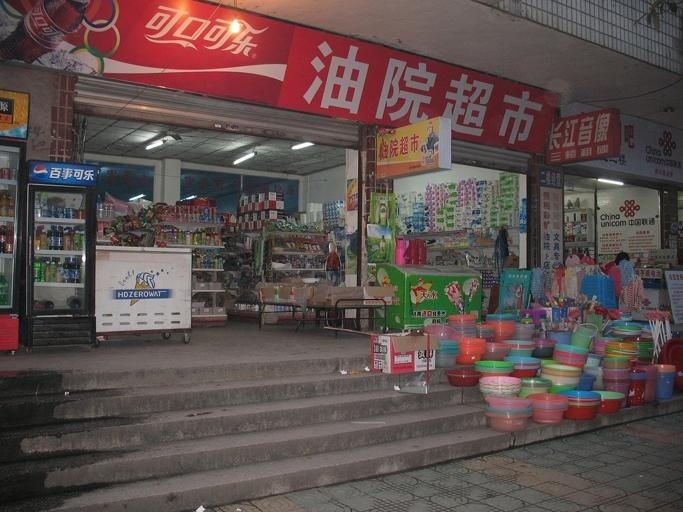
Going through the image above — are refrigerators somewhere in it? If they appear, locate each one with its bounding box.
[95,245,193,344]
[372,261,484,333]
[0,88,31,358]
[19,158,99,353]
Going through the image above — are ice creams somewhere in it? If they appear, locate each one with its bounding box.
[130,271,155,305]
[468,280,480,304]
[444,282,464,312]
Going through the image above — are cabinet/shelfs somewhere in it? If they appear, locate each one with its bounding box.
[224,229,337,320]
[154,219,228,326]
[402,227,520,273]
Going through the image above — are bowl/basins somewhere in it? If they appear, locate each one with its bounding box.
[420,312,683,433]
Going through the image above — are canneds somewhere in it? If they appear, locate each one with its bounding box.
[1,168,10,179]
[10,168,16,179]
[33,205,86,219]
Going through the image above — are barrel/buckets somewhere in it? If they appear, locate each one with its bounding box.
[571,320,599,348]
[656,364,676,400]
[628,370,649,406]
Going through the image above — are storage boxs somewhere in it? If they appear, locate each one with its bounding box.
[371,333,437,375]
[261,285,394,306]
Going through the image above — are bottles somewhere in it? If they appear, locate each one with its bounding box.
[0,0,91,65]
[66,295,82,309]
[96,199,114,219]
[160,228,202,245]
[44,300,54,309]
[33,224,81,284]
[324,240,343,330]
[379,235,386,261]
[379,199,388,227]
[1,193,8,218]
[395,238,427,265]
[0,224,13,254]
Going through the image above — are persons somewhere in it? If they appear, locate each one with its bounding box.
[419,122,438,152]
